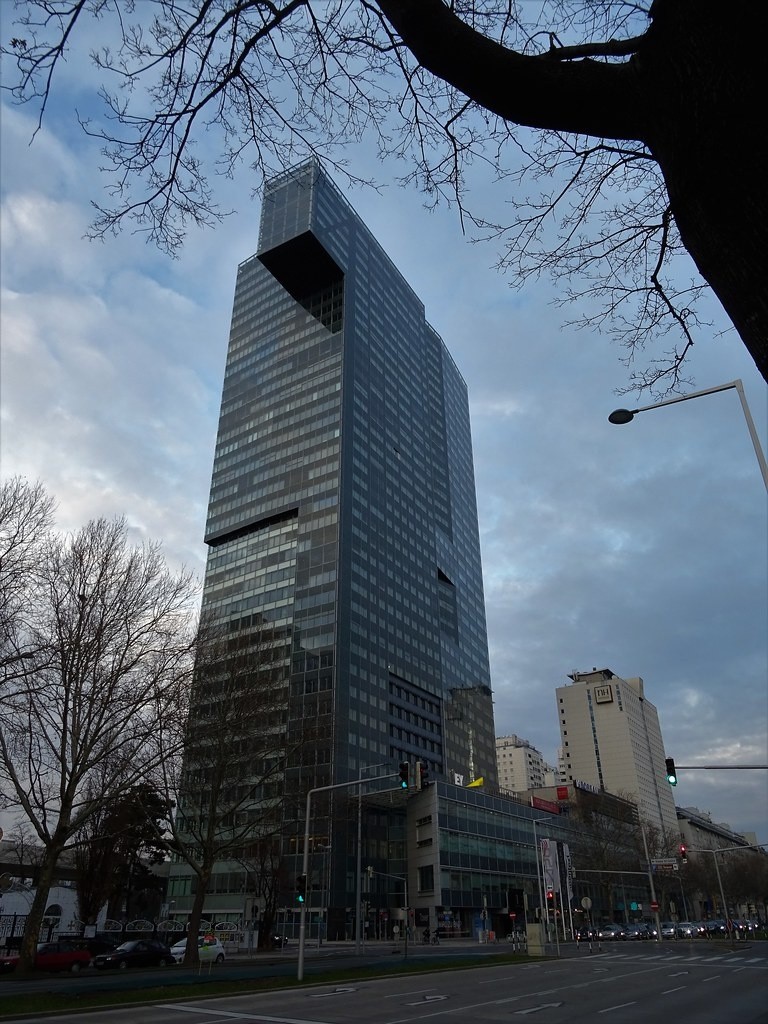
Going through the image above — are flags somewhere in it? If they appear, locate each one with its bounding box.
[563,843,573,900]
[548,841,561,892]
[541,838,554,892]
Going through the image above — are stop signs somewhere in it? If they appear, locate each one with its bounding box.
[650,901,660,910]
[507,911,516,924]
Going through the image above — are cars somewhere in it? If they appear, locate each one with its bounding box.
[91,938,170,971]
[171,934,224,967]
[2,940,90,974]
[575,919,759,941]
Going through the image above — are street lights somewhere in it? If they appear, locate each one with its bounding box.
[609,376,768,540]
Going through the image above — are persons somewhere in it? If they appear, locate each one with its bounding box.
[432,927,440,945]
[422,926,431,945]
[591,927,597,941]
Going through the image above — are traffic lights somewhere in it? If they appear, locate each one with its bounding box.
[679,844,687,859]
[414,761,430,792]
[548,889,554,900]
[296,875,307,905]
[663,756,676,787]
[398,762,410,793]
